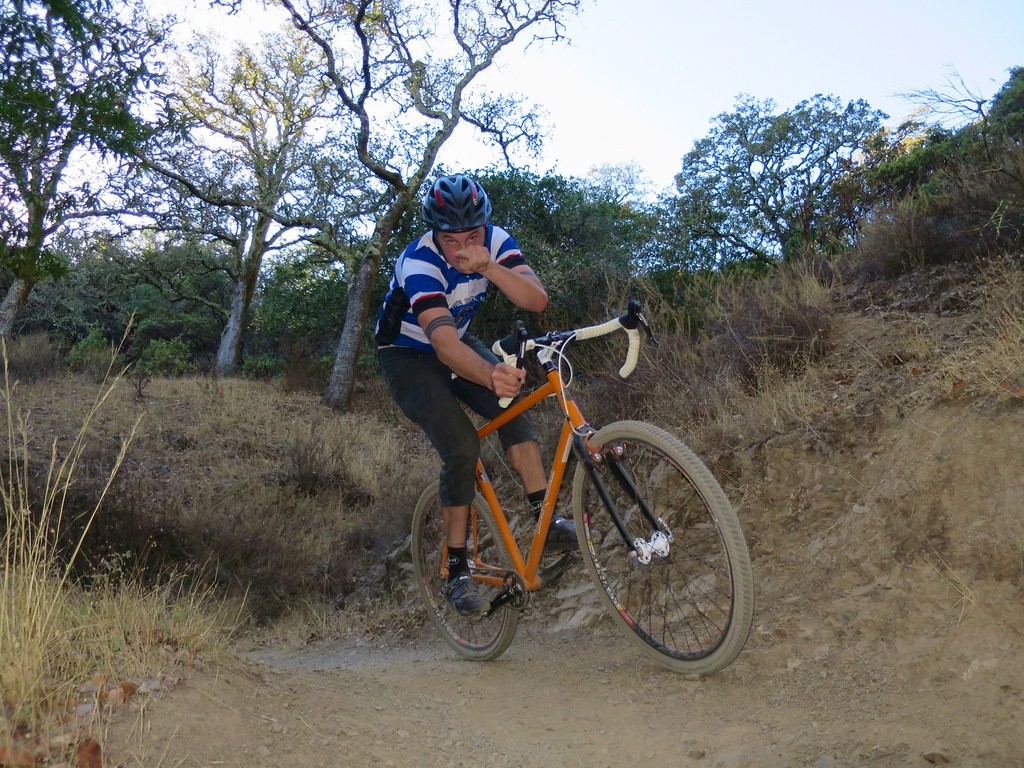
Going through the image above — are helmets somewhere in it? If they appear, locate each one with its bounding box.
[421,174,492,233]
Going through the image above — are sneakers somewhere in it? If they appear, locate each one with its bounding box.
[541,516,602,553]
[444,571,492,617]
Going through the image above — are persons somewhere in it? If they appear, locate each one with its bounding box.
[373,173,603,616]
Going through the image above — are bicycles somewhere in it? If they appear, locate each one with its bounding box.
[409,298,753,678]
